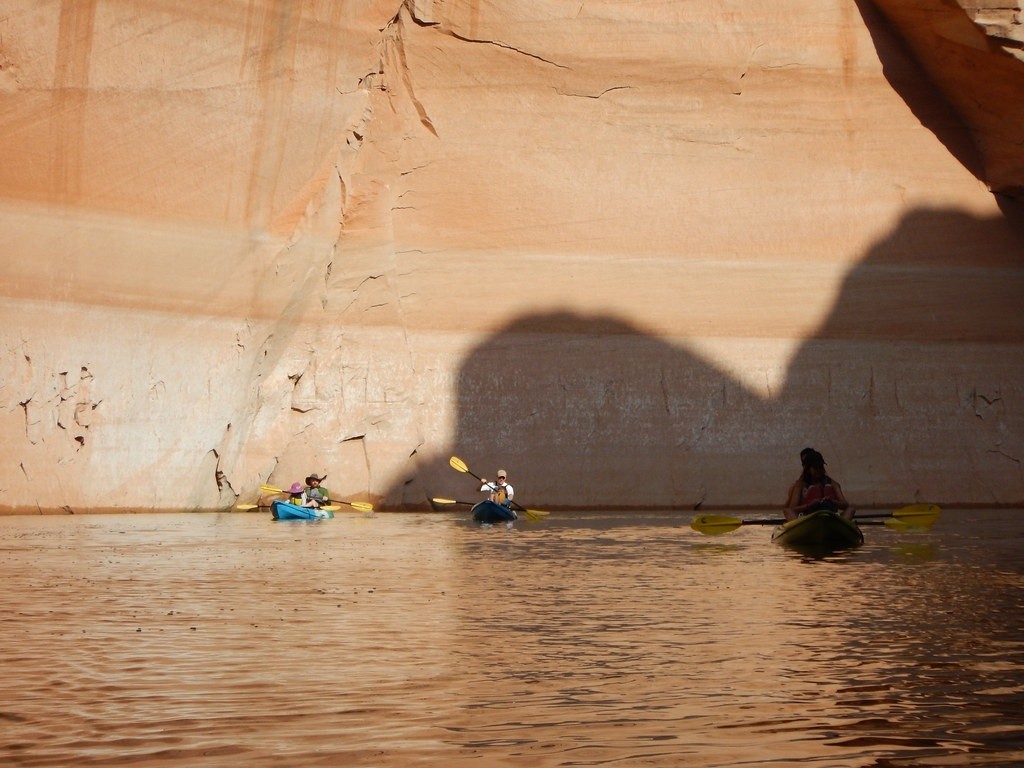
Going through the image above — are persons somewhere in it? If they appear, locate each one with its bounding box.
[284,474,331,509]
[783,447,856,523]
[476,469,514,505]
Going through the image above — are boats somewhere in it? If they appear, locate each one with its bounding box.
[269,500,334,520]
[469,499,518,522]
[769,509,866,555]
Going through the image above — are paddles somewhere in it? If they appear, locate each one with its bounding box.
[448,455,544,524]
[695,502,944,537]
[258,484,374,513]
[432,497,551,517]
[690,512,935,533]
[236,504,342,511]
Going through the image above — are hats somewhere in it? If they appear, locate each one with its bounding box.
[305,474,322,486]
[497,469,507,479]
[289,482,304,494]
[804,451,823,466]
